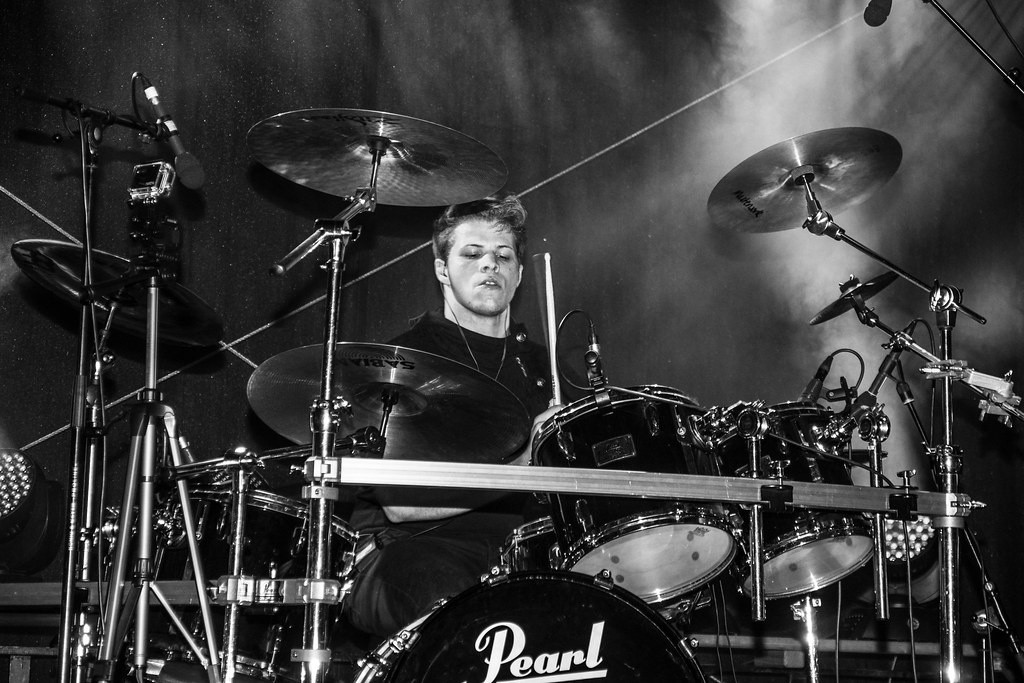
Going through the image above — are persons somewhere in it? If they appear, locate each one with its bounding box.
[328,192,580,648]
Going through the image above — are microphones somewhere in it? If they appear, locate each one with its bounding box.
[800,354,834,403]
[588,330,608,387]
[855,320,916,409]
[139,77,205,189]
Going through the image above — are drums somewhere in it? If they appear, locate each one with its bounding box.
[128,488,361,683]
[349,566,709,683]
[717,400,876,601]
[532,384,741,606]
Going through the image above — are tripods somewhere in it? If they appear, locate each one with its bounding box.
[97,275,226,683]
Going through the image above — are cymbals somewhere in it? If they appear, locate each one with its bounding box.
[245,106,510,207]
[705,125,904,234]
[11,237,225,348]
[245,340,532,464]
